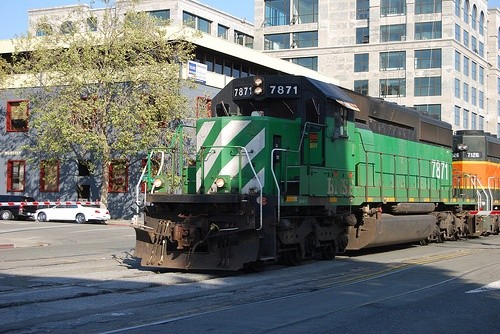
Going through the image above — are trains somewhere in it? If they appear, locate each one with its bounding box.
[122,71,500,274]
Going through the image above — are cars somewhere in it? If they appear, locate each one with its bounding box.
[0,194,37,221]
[33,201,111,224]
